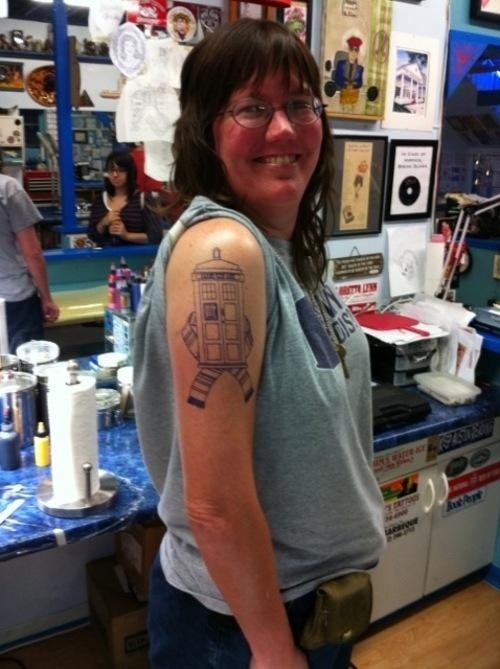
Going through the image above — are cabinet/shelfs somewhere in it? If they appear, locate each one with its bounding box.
[365,415,500,627]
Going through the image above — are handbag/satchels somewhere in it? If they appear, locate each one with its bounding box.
[301,572,372,650]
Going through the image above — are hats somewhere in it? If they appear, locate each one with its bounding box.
[347,37,362,47]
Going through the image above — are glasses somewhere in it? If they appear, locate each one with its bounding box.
[218,96,327,128]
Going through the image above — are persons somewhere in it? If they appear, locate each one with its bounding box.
[334,36,364,115]
[130,15,387,669]
[0,175,61,355]
[89,149,162,248]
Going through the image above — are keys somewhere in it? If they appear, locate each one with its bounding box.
[336,345,350,379]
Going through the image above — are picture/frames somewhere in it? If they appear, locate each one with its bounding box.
[320,133,388,239]
[317,0,394,123]
[382,136,439,224]
[381,27,441,132]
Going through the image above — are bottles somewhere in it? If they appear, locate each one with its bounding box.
[423,235,444,295]
[31,421,50,467]
[0,408,20,470]
[106,257,134,314]
[0,339,144,452]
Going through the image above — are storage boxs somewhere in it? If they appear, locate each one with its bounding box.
[85,551,150,669]
[120,519,167,600]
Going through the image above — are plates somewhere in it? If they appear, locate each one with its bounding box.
[24,64,57,108]
